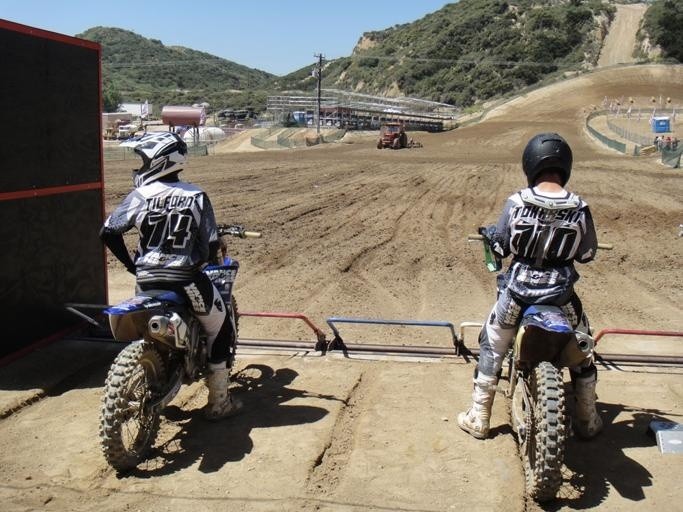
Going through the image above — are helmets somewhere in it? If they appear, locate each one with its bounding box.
[522,132,573,187]
[131,131,187,187]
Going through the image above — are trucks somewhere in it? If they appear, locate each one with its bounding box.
[119,125,138,139]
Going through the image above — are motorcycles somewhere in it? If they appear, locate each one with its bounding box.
[467,234,614,500]
[98,224,261,470]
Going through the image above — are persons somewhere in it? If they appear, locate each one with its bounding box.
[457,133,603,438]
[100,131,243,421]
[654,135,678,153]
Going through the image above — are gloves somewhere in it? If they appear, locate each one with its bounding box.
[482,225,497,243]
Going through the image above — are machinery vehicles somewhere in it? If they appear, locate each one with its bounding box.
[377,123,407,149]
[103,120,130,140]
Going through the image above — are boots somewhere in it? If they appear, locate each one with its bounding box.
[206,370,243,420]
[457,375,498,439]
[574,373,603,438]
